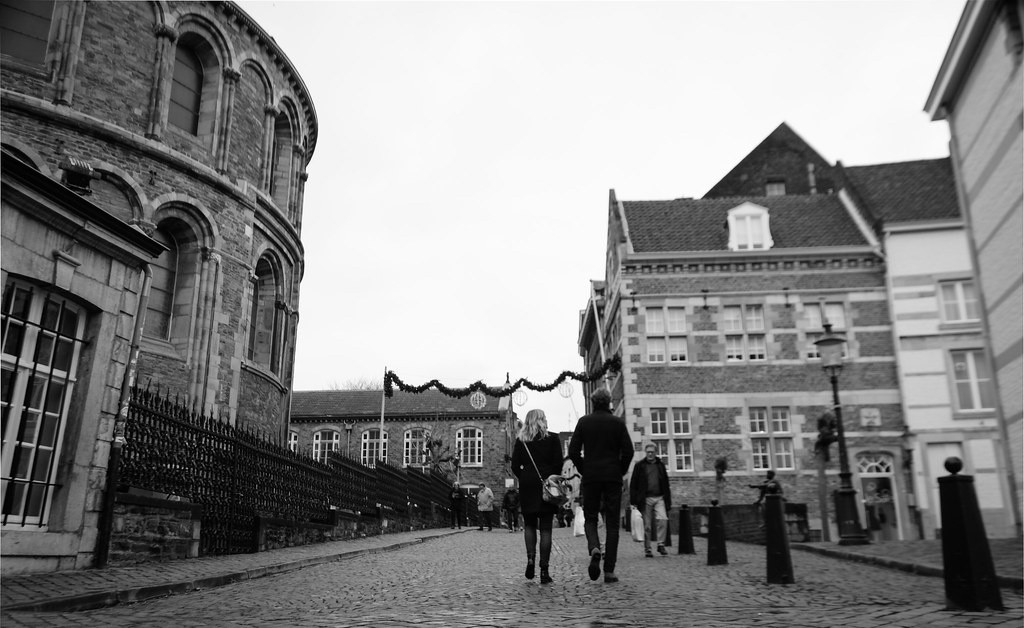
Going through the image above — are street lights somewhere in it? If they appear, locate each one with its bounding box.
[812,315,872,545]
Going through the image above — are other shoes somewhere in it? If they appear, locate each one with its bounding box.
[587,549,620,584]
[509,527,517,533]
[458,525,462,530]
[477,526,483,531]
[524,554,554,583]
[451,525,455,529]
[488,526,492,531]
[645,548,653,557]
[657,545,669,555]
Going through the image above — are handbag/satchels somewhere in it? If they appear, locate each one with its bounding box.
[542,474,570,500]
[630,506,646,543]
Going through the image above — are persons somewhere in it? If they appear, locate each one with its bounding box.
[477,484,495,531]
[630,444,671,558]
[502,484,573,532]
[568,387,635,582]
[449,482,464,529]
[511,409,563,584]
